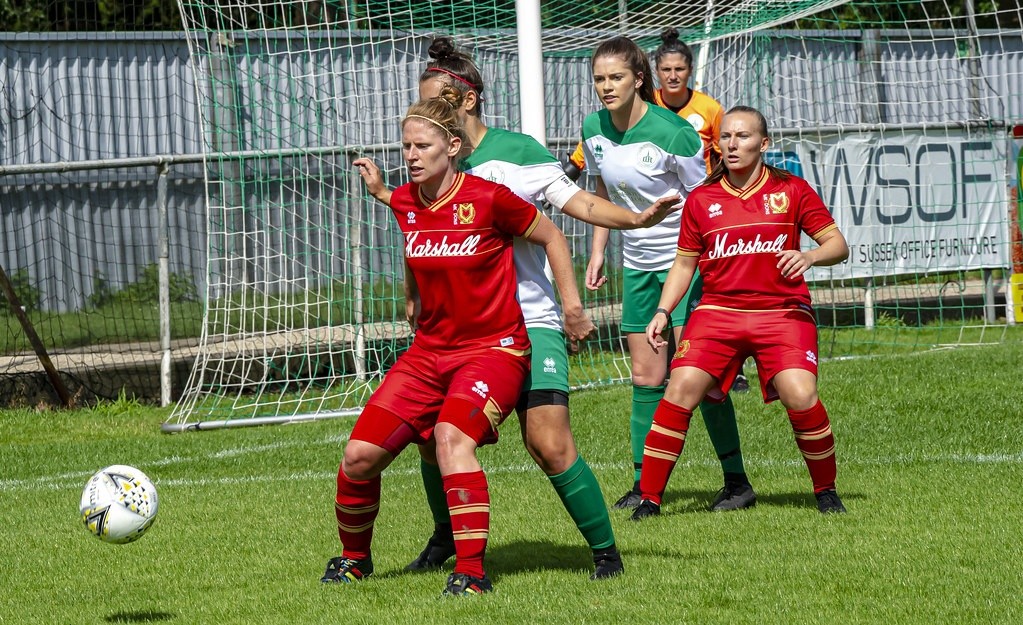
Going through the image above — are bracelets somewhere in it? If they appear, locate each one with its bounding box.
[653,308,672,324]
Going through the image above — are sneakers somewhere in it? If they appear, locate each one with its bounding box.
[321,549,374,583]
[613,490,642,509]
[442,572,492,598]
[628,498,660,523]
[405,531,456,572]
[591,551,625,582]
[816,489,846,514]
[707,485,756,512]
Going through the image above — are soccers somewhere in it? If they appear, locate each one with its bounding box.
[78,463,160,546]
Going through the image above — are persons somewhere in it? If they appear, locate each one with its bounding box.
[541,25,751,394]
[625,106,850,524]
[353,39,683,584]
[322,84,601,595]
[581,37,758,512]
